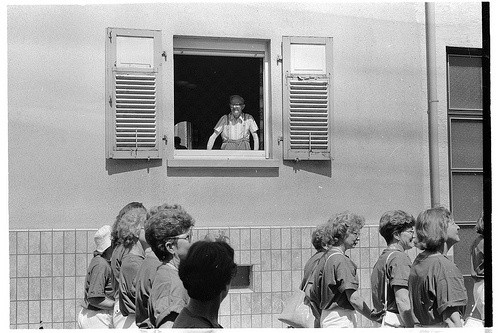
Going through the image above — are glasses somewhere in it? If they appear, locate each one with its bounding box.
[229,103,244,106]
[169,235,192,242]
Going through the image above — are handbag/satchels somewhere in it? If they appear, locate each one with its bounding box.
[463,315,485,328]
[278,289,319,329]
[380,312,405,329]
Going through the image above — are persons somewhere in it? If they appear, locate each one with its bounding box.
[115,206,151,328]
[406,206,468,328]
[206,95,260,150]
[370,209,418,328]
[174,135,187,150]
[170,238,239,328]
[469,208,485,324]
[108,201,147,329]
[135,201,188,329]
[299,221,328,328]
[75,224,115,329]
[312,212,381,327]
[145,207,195,328]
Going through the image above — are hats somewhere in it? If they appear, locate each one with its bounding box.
[94,225,113,253]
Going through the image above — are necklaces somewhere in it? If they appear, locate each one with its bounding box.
[163,261,181,272]
